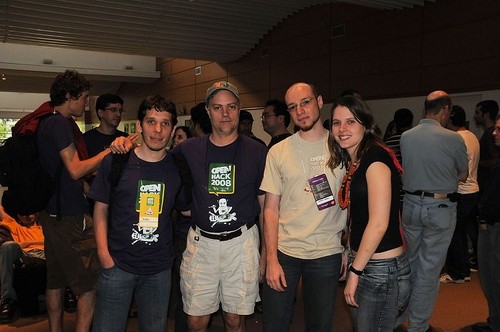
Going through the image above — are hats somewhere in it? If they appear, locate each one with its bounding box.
[205,81,241,104]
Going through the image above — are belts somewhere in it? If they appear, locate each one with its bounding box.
[192,223,255,241]
[405,190,457,198]
[478,216,499,225]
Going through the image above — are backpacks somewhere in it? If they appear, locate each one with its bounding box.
[0,115,60,215]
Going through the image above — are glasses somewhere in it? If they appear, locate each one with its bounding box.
[102,107,123,114]
[287,95,317,112]
[260,115,276,121]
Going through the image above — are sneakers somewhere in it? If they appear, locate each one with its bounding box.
[472,324,495,332]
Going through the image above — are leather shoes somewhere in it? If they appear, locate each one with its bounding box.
[0,298,21,322]
[64,287,76,313]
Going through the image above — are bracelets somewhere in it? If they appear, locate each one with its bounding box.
[350,265,364,276]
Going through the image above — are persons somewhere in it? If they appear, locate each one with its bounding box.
[326,93,413,332]
[393,90,469,332]
[470,100,499,272]
[260,99,293,154]
[109,81,267,332]
[86,95,191,332]
[382,108,414,220]
[36,69,139,332]
[169,102,268,150]
[259,82,351,332]
[0,204,77,322]
[473,114,500,332]
[83,94,129,219]
[439,105,479,284]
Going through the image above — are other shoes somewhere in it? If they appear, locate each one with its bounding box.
[468,255,477,271]
[244,314,254,319]
[255,306,263,313]
[439,272,470,283]
